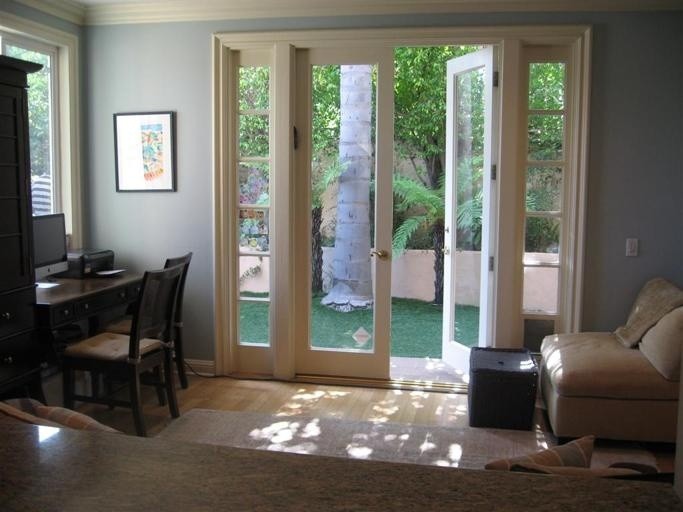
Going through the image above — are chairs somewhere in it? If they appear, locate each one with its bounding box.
[108,251,193,406]
[541,277,682,447]
[61,264,185,437]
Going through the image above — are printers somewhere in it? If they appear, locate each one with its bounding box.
[51,247,115,278]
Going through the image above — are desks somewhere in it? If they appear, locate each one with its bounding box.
[35,271,144,412]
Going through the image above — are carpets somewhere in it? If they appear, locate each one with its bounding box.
[157,410,660,473]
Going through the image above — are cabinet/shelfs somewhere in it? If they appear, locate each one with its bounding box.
[0,54,45,407]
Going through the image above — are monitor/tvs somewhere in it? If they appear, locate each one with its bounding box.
[32,213,69,285]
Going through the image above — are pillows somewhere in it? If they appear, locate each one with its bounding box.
[5,398,125,435]
[484,434,595,472]
[511,463,641,477]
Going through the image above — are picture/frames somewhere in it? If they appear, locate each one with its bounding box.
[114,110,176,192]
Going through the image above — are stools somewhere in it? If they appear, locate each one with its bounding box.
[468,346,538,429]
